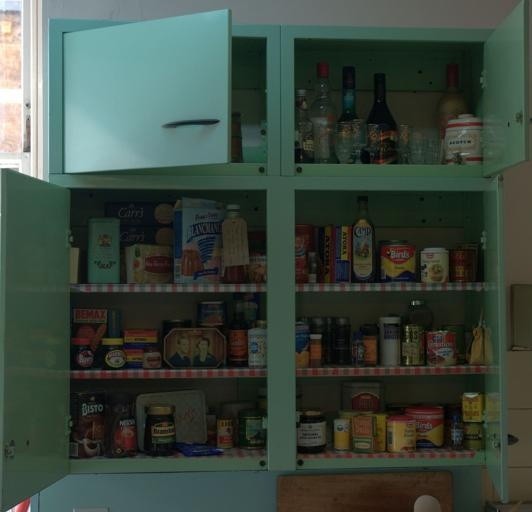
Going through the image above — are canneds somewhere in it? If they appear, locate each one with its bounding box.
[239,409,266,450]
[377,239,476,285]
[463,420,483,450]
[71,337,127,370]
[227,320,255,367]
[296,410,328,453]
[216,416,234,449]
[401,324,426,366]
[160,318,191,368]
[386,415,417,453]
[425,330,458,366]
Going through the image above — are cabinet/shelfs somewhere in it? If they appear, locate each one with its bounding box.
[43,2,532,177]
[31,471,485,511]
[0,166,509,510]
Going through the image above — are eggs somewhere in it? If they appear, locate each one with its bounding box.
[414,495,441,512]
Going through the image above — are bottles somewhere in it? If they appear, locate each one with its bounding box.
[307,250,323,283]
[450,412,464,447]
[351,192,377,283]
[295,40,445,164]
[221,202,250,283]
[310,297,434,366]
